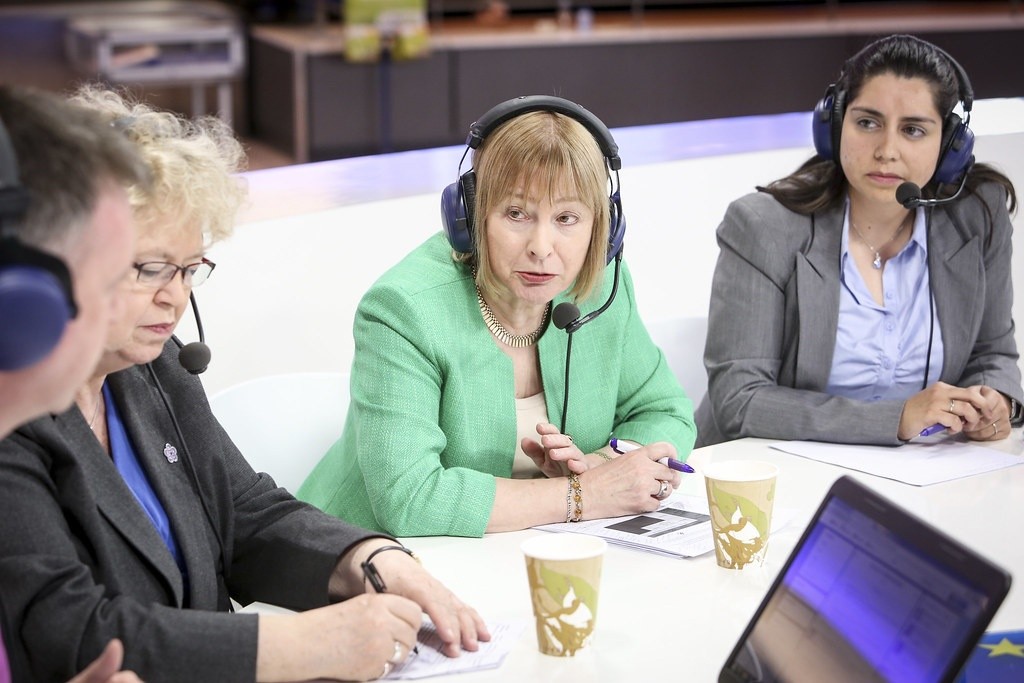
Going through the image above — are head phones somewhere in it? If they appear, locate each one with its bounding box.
[0,117,79,373]
[441,94,626,268]
[812,32,974,184]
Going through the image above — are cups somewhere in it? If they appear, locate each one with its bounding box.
[703,457,782,570]
[523,533,606,657]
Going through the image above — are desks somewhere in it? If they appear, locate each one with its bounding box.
[251,8,1024,161]
[224,439,1021,683]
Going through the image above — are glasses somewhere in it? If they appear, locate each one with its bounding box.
[132,259,214,288]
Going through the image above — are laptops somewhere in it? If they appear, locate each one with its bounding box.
[718,475,1013,683]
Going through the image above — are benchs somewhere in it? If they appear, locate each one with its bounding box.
[109,98,1024,515]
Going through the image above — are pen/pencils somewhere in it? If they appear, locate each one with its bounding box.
[609,438,697,473]
[361,562,420,655]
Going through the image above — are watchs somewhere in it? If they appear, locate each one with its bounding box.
[1009,398,1016,420]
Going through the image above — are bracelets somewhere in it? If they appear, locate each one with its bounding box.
[591,451,613,462]
[567,473,583,523]
[363,545,421,583]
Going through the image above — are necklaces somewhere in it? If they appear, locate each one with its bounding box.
[849,216,908,269]
[90,395,100,429]
[471,264,549,348]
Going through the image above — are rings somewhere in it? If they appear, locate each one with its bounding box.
[391,641,401,663]
[379,663,390,677]
[993,424,997,434]
[656,479,667,498]
[949,399,954,413]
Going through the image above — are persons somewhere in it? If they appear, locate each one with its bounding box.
[694,35,1024,447]
[0,80,491,683]
[295,94,698,538]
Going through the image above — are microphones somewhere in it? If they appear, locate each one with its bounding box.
[896,155,975,210]
[553,243,624,335]
[179,289,212,376]
